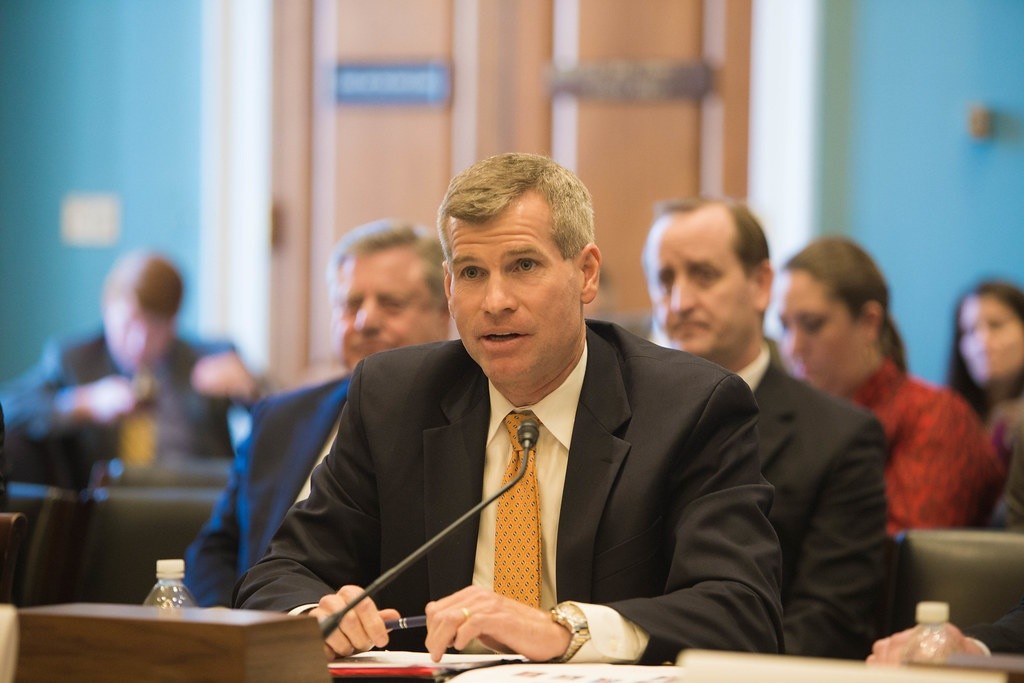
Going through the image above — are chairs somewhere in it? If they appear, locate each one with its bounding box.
[0,455,1023,658]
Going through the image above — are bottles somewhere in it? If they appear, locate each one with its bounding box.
[904,603,965,663]
[139,557,199,611]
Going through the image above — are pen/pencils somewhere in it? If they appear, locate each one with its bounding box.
[385,616,426,630]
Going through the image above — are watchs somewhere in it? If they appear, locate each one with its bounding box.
[551,602,591,663]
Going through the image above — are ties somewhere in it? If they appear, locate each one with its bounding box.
[122,377,156,469]
[495,409,541,613]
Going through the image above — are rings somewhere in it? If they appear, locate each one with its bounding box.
[460,607,469,620]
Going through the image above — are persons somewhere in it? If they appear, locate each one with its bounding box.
[0,254,276,487]
[641,195,889,660]
[949,281,1024,534]
[178,218,449,607]
[773,238,1005,542]
[234,151,784,666]
